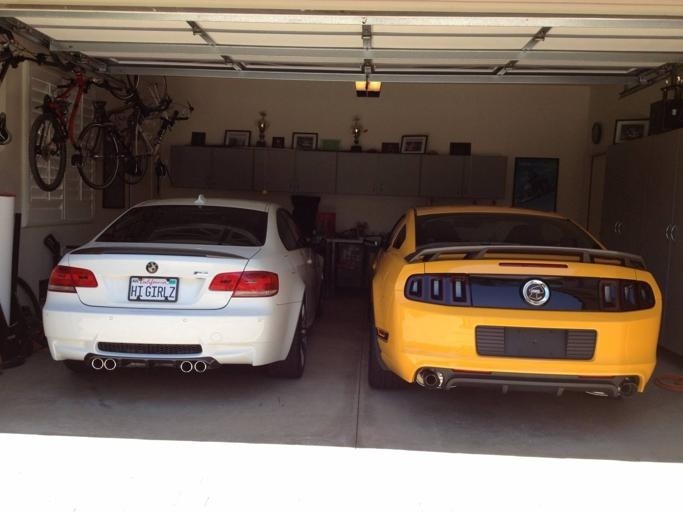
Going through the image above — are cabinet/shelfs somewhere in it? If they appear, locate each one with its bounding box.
[601,129,682,360]
[173,144,507,201]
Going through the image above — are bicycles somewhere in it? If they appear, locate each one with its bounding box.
[0,24,190,190]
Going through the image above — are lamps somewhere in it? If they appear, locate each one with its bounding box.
[355,81,381,97]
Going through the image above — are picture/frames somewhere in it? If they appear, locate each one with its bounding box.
[400,134,427,154]
[291,131,318,150]
[224,130,251,145]
[613,117,649,144]
